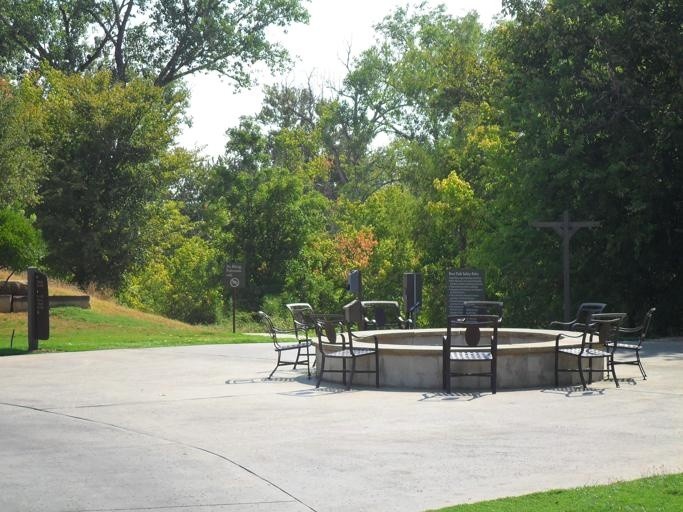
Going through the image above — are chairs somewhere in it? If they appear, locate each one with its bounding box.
[441,315,499,395]
[308,312,380,392]
[552,311,626,393]
[602,307,656,388]
[548,301,607,333]
[284,302,342,370]
[257,310,312,378]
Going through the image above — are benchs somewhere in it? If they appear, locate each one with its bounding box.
[359,300,412,331]
[455,300,503,328]
